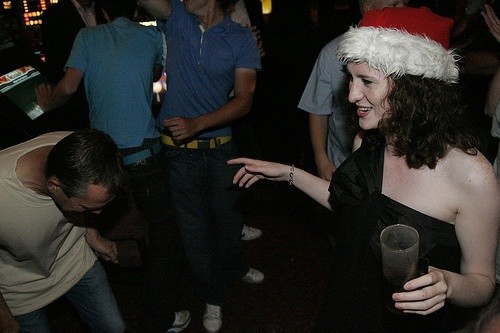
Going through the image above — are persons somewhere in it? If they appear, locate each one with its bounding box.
[226,5,500,333]
[296,0,500,333]
[35,0,265,333]
[0,128,127,333]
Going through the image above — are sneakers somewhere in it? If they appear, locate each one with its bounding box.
[202,303,222,332]
[242,268,264,284]
[167,309,191,333]
[242,225,262,241]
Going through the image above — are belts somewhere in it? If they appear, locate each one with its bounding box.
[162,136,233,149]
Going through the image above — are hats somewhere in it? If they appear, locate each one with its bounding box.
[335,7,460,84]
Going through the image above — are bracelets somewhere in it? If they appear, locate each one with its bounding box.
[287,164,295,187]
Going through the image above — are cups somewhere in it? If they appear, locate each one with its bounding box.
[380,224,419,313]
